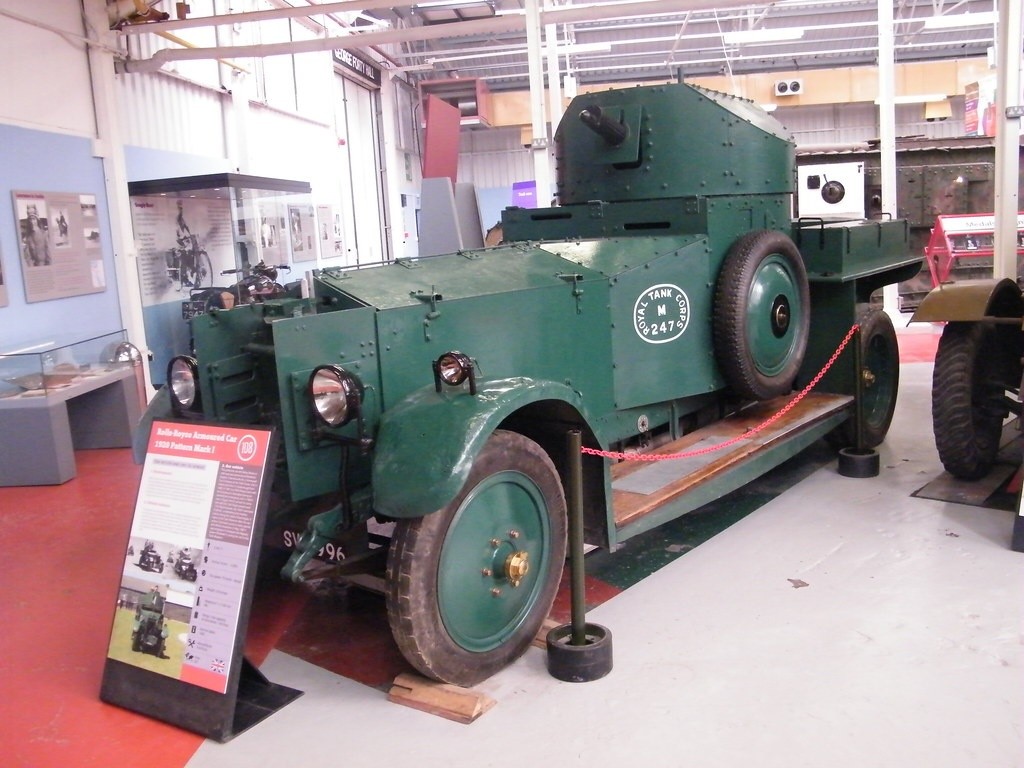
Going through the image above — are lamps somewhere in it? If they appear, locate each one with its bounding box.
[542,42,611,58]
[722,26,805,44]
[923,0,999,30]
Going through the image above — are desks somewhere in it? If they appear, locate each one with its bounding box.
[0,367,143,488]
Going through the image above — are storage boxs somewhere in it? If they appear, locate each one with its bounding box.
[129,173,321,389]
[0,328,133,399]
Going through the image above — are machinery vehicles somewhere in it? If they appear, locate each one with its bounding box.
[133,68,930,691]
